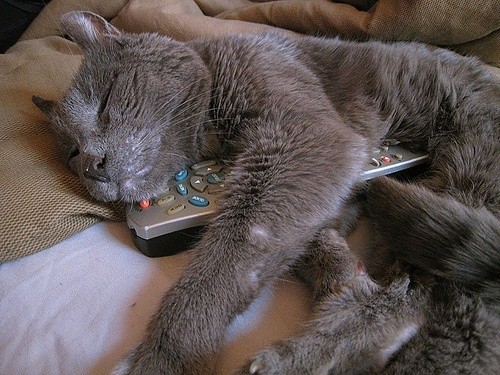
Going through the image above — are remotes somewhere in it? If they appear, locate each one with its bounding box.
[123,145,429,259]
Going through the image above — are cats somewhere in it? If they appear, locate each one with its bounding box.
[30,8,500,374]
